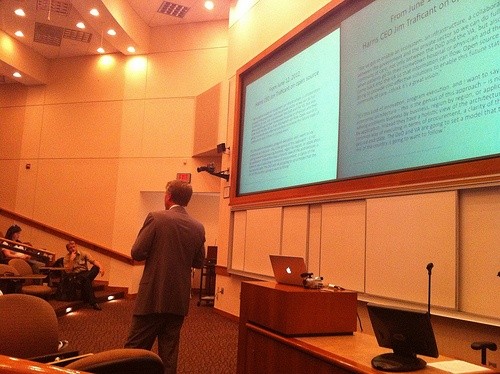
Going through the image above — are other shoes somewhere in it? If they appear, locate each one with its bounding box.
[93,303,101,310]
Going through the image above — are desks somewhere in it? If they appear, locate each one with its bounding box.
[0,273,48,293]
[40,265,65,285]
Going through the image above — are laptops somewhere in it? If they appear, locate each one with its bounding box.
[269,254,307,286]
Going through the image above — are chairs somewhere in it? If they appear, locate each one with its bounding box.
[0,259,53,296]
[64,349,165,374]
[0,293,59,357]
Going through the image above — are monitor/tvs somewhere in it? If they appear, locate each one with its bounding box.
[366,303,439,372]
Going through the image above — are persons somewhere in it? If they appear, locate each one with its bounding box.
[124,180,206,374]
[63,240,104,311]
[4,224,23,244]
